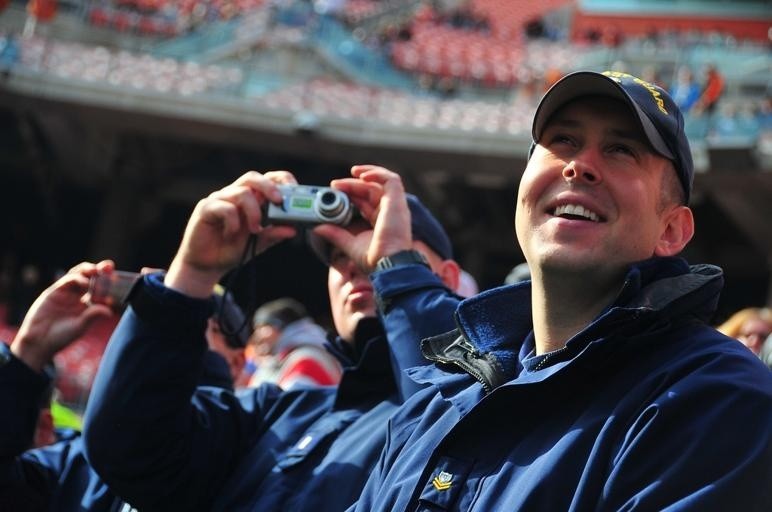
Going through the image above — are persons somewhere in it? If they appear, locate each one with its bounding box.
[347,71,772,512]
[79,163,468,512]
[0,260,283,512]
[246,297,345,391]
[718,307,772,356]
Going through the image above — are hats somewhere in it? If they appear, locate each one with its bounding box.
[250,299,309,324]
[528,71,695,208]
[306,193,454,268]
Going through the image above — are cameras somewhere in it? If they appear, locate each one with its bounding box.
[268,183,353,228]
[88,270,140,312]
